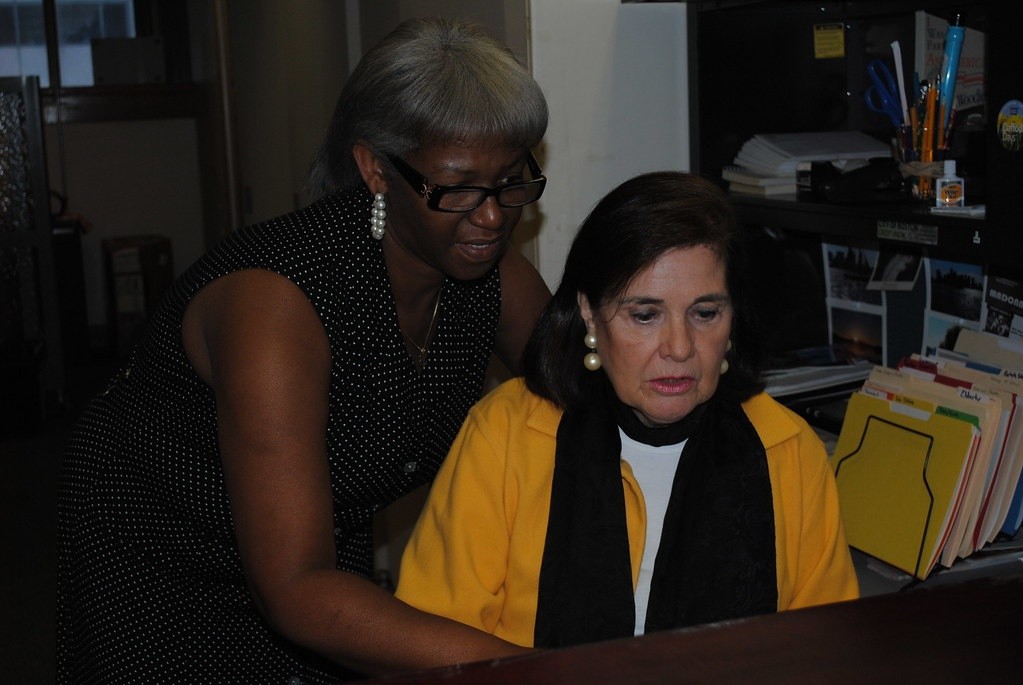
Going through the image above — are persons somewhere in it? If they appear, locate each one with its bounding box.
[53,16,554,685]
[393,172,860,650]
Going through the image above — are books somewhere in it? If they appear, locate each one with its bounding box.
[722,130,893,202]
[756,327,1023,580]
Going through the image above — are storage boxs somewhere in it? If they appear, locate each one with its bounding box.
[102,235,172,348]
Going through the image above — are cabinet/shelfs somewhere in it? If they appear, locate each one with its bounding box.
[686,0,1023,440]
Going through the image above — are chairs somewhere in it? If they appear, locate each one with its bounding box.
[1,75,84,409]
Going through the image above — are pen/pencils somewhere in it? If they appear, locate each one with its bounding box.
[891,15,965,202]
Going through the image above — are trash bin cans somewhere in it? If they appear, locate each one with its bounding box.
[50,219,91,362]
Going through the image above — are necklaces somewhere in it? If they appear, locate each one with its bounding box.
[400,288,444,370]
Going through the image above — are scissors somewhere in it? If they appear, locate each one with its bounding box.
[863,56,903,128]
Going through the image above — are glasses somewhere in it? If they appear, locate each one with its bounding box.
[377,150,547,213]
